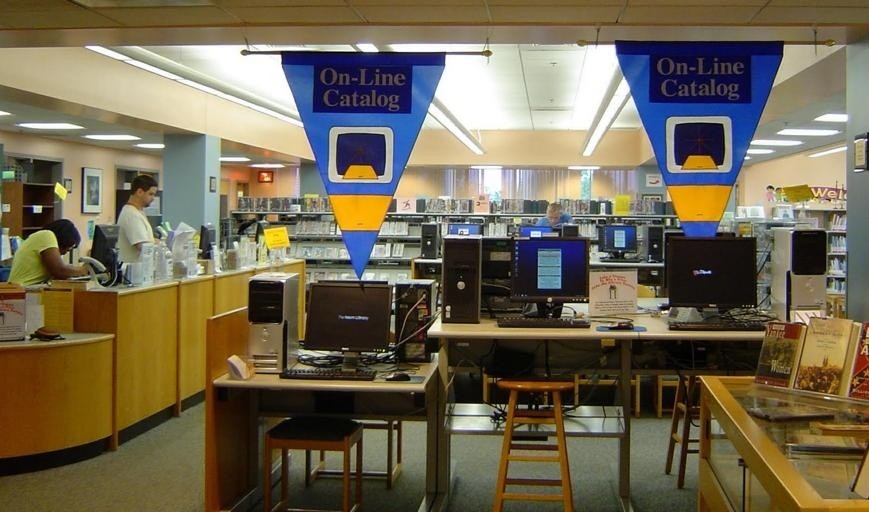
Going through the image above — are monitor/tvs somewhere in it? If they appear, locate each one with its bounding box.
[664,232,736,289]
[304,284,393,369]
[88,224,119,285]
[482,236,510,278]
[603,225,637,258]
[199,223,216,260]
[447,223,483,236]
[510,237,589,319]
[519,225,561,238]
[598,226,605,252]
[668,236,757,323]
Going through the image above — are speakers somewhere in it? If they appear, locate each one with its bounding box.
[226,354,250,380]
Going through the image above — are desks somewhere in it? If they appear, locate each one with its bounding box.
[590,261,668,289]
[203,305,437,512]
[425,310,785,512]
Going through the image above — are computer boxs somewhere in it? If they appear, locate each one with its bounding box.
[647,225,663,262]
[394,280,436,363]
[441,235,482,323]
[249,272,300,374]
[561,223,579,237]
[775,228,827,306]
[421,222,440,258]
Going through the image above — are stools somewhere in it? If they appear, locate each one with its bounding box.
[826,293,845,319]
[837,298,846,320]
[573,374,642,419]
[481,367,549,410]
[665,372,702,488]
[655,374,702,419]
[303,419,404,489]
[497,379,578,512]
[268,417,363,512]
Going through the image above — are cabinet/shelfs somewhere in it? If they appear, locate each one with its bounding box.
[793,209,848,293]
[443,213,679,243]
[300,210,438,312]
[410,256,443,289]
[736,218,819,309]
[0,180,55,245]
[231,209,298,264]
[698,376,868,512]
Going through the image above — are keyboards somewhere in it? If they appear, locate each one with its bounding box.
[601,259,641,263]
[497,316,591,329]
[280,368,378,381]
[670,322,766,331]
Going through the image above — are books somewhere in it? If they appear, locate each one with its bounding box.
[828,257,846,276]
[829,213,846,231]
[827,278,845,294]
[828,235,847,254]
[785,434,864,460]
[755,321,807,389]
[794,316,862,398]
[847,322,869,403]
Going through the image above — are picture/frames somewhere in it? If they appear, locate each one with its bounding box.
[208,177,217,193]
[853,133,869,168]
[645,172,663,188]
[64,178,72,193]
[80,166,102,213]
[642,193,664,205]
[257,170,274,183]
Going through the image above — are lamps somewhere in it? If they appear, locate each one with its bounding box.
[578,54,646,158]
[351,43,487,156]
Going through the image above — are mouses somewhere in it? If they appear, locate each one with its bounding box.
[657,303,667,309]
[609,321,632,330]
[386,372,410,381]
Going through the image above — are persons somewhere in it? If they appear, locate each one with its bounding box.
[536,203,576,230]
[115,174,161,271]
[6,218,88,292]
